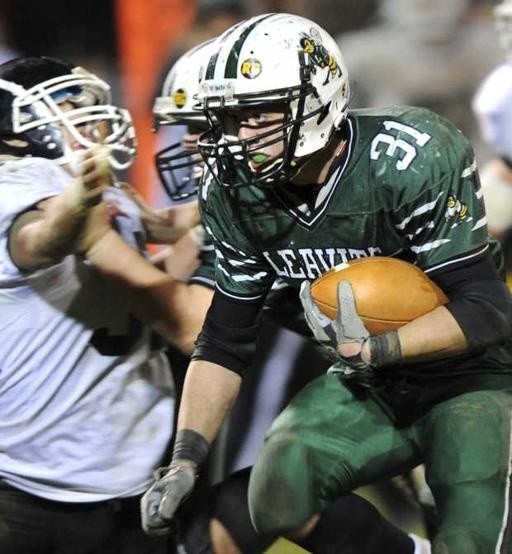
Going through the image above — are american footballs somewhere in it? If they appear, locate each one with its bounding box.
[306,256,451,336]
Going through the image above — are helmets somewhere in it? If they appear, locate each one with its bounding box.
[191,12,352,191]
[150,36,219,201]
[1,53,138,172]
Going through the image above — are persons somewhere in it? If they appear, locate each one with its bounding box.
[0,2,512,554]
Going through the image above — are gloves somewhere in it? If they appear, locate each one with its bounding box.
[298,279,404,374]
[139,459,199,539]
